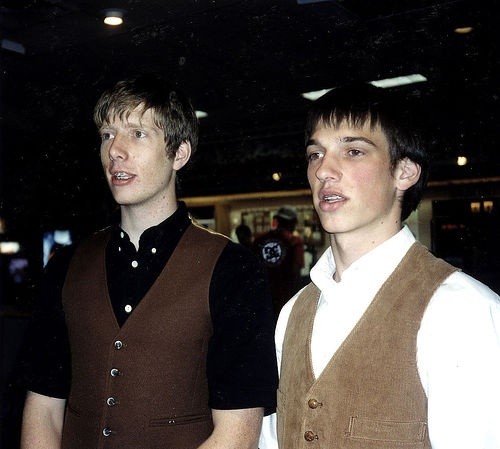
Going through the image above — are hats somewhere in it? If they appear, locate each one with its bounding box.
[273,208,296,219]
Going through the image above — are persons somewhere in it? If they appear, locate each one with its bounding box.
[231,207,321,279]
[20,78,278,449]
[258,83,500,449]
